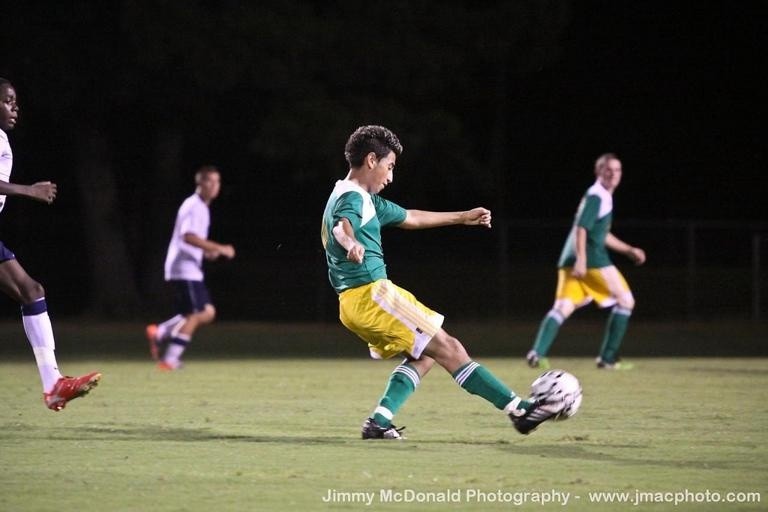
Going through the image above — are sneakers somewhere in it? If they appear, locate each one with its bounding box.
[527,349,551,371]
[145,322,187,373]
[43,370,105,414]
[593,355,637,372]
[360,417,410,441]
[504,391,574,437]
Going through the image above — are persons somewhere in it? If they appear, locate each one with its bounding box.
[0,78,105,412]
[144,165,236,371]
[527,150,648,371]
[320,124,571,439]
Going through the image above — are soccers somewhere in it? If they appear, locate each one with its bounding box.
[530,368,582,421]
